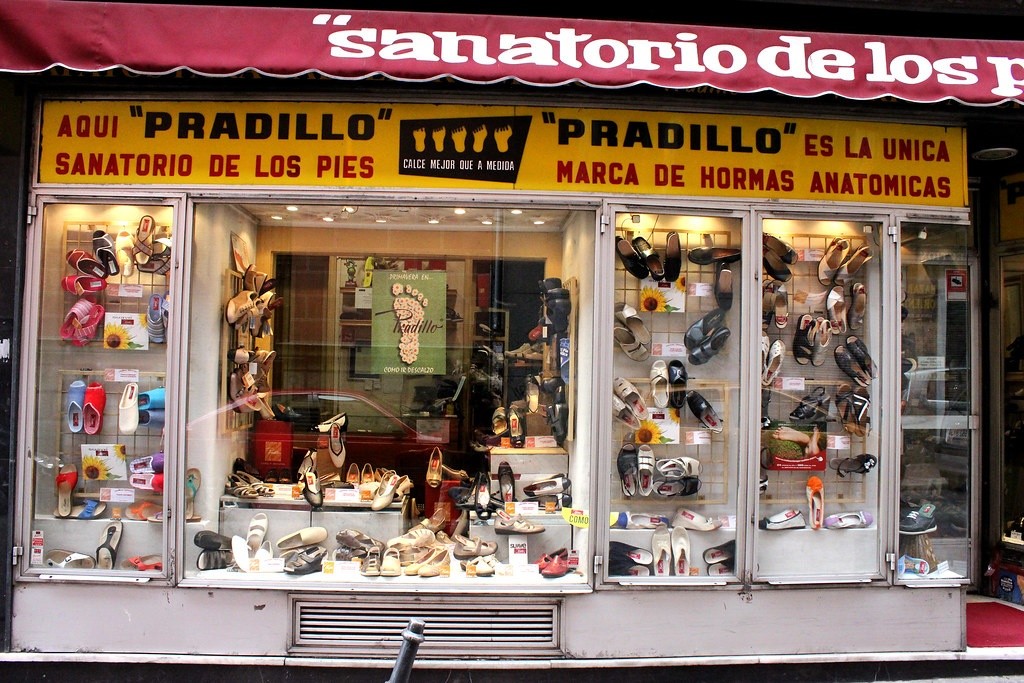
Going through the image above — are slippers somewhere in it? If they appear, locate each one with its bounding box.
[43,216,171,570]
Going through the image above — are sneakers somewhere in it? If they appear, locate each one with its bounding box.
[899,510,937,534]
[936,500,967,529]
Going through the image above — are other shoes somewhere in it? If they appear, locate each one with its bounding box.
[902,357,917,372]
[228,266,302,419]
[902,289,909,321]
[427,277,581,577]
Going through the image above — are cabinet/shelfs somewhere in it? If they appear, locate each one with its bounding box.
[470,308,510,441]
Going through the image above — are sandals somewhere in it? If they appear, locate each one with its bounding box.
[758,232,879,530]
[608,232,740,577]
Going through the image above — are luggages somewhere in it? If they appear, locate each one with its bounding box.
[182,413,451,576]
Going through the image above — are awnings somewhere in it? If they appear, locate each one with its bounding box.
[0,2,1024,112]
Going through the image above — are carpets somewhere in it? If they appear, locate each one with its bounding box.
[967,601,1024,648]
